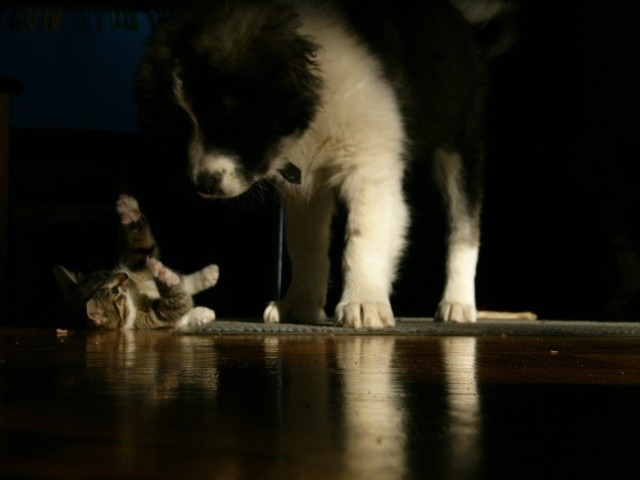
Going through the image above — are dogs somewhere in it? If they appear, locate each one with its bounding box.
[141,0,514,331]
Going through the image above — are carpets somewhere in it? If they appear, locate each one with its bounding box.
[174,320,640,343]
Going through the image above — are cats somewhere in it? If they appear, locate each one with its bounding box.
[50,192,221,335]
